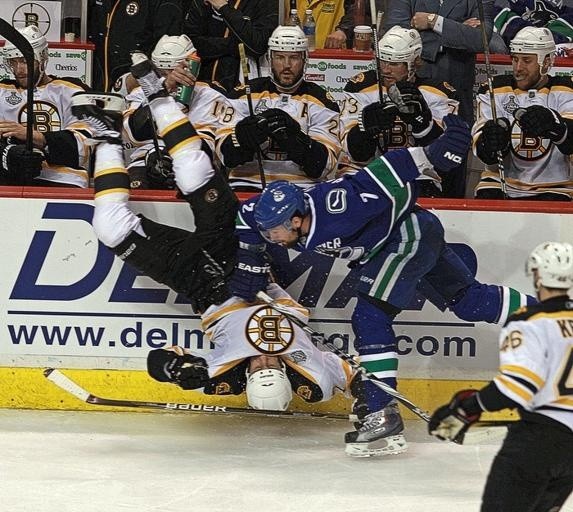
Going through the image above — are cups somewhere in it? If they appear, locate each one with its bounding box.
[64,32,74,42]
[353,25,373,51]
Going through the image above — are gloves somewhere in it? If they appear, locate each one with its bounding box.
[168,354,208,389]
[231,251,270,301]
[428,390,480,444]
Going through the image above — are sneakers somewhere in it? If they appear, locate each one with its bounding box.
[347,405,404,442]
[127,52,168,98]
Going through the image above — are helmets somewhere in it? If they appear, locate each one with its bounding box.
[377,25,423,80]
[3,25,49,70]
[527,242,573,289]
[153,34,197,72]
[267,24,308,70]
[245,360,291,411]
[509,26,556,75]
[254,180,309,231]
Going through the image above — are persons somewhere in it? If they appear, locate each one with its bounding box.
[427,240,570,512]
[68,62,354,414]
[252,113,538,442]
[0,0,570,203]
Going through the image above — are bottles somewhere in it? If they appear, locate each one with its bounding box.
[175,55,201,102]
[289,8,317,51]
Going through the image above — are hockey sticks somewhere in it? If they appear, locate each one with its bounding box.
[369,0,388,154]
[43,368,362,423]
[0,17,34,153]
[125,93,173,190]
[254,291,507,445]
